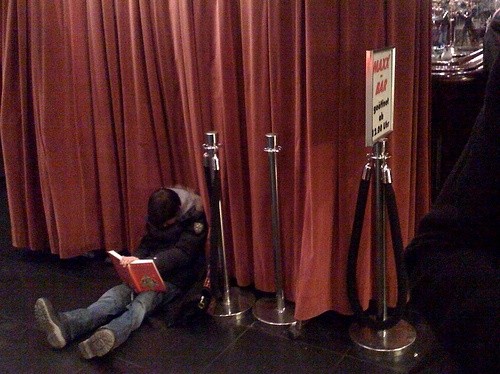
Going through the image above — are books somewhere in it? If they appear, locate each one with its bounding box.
[108,249,168,293]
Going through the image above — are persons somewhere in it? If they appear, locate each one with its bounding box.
[34,184,209,359]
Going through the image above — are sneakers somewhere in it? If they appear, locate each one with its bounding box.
[75,329,115,360]
[34,298,72,349]
